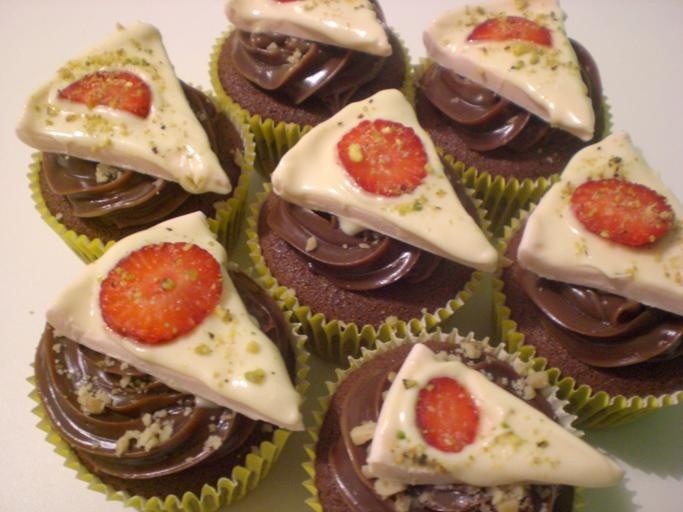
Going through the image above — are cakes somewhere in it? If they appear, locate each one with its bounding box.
[15,0,683,512]
